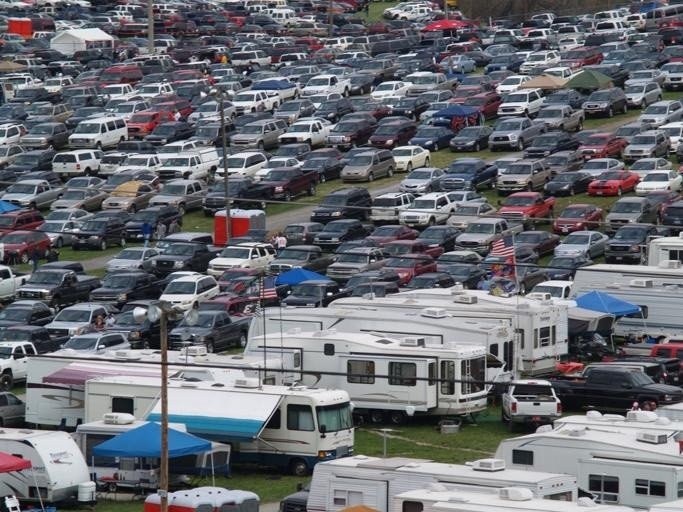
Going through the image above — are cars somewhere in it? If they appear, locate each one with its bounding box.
[0,1,682,512]
[275,477,308,510]
[551,363,683,416]
[497,376,561,430]
[601,337,682,383]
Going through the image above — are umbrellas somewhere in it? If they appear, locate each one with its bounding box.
[420,18,469,43]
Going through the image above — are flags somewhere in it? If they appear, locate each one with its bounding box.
[490,257,516,281]
[259,274,277,300]
[490,235,514,257]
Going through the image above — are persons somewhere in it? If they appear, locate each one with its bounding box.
[110,47,137,63]
[270,232,279,245]
[195,112,204,134]
[31,244,42,273]
[154,220,167,240]
[430,51,437,71]
[94,314,106,329]
[178,112,187,123]
[446,53,454,74]
[273,233,288,252]
[256,101,267,112]
[103,313,116,328]
[655,38,666,53]
[45,245,62,263]
[476,273,487,290]
[171,108,182,122]
[167,218,181,234]
[187,49,228,66]
[141,218,153,248]
[134,311,154,350]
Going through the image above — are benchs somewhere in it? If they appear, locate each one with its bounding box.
[104,480,143,495]
